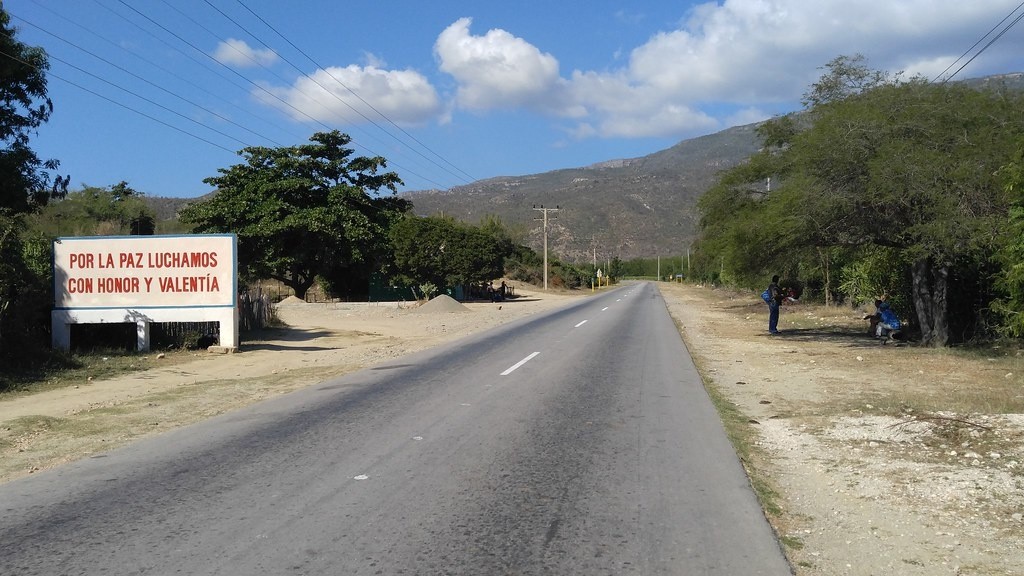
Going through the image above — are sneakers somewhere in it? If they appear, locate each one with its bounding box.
[768,331,783,336]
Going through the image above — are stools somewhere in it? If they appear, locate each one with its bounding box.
[883,329,901,344]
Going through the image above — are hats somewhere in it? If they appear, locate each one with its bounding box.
[879,302,889,307]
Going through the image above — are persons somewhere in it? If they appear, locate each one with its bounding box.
[767,275,793,335]
[863,300,900,340]
[471,281,509,302]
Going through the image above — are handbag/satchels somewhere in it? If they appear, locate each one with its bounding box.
[760,288,772,304]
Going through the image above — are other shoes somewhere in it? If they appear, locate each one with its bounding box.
[872,335,880,338]
